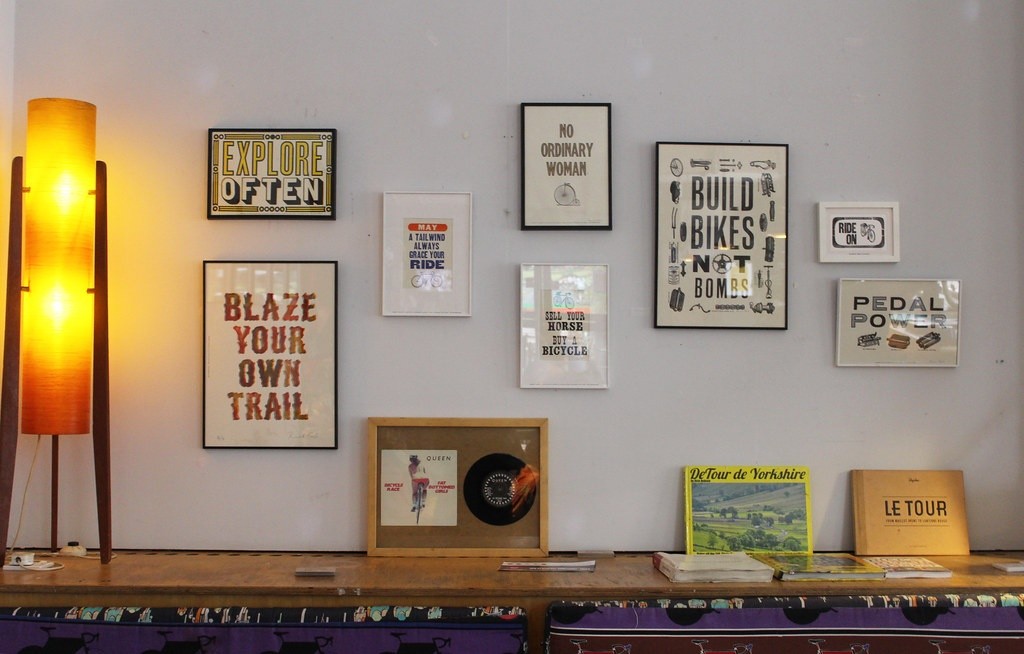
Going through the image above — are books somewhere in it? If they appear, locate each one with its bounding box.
[652,551,774,582]
[863,556,954,579]
[991,561,1024,573]
[682,465,814,556]
[848,469,971,557]
[751,552,887,581]
[296,565,336,577]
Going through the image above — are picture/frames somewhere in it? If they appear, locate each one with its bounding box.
[520,263,611,390]
[382,190,473,316]
[367,417,549,558]
[656,141,788,332]
[836,278,959,368]
[521,103,613,231]
[203,260,338,450]
[208,127,337,222]
[818,201,901,264]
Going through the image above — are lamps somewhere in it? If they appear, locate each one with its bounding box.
[0,98,113,569]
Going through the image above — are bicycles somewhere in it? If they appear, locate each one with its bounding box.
[414,482,424,524]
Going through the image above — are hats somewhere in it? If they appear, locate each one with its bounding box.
[410,455,417,458]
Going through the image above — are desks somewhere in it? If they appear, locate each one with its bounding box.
[0,547,1024,654]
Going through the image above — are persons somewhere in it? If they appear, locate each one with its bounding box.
[407,454,430,511]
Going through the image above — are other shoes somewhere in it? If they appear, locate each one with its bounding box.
[420,504,425,509]
[411,506,416,512]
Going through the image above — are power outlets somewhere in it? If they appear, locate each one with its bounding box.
[3,561,55,570]
